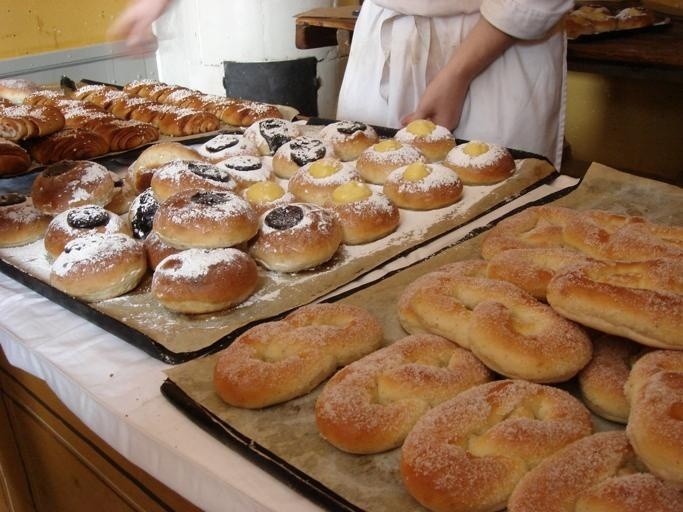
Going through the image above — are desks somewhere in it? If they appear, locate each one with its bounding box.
[0,174,582,512]
[295,1,683,77]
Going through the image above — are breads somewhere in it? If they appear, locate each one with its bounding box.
[564,1,655,38]
[1,113,517,314]
[214,204,683,511]
[0,76,285,181]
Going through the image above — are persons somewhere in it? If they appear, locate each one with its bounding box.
[105,0,578,172]
